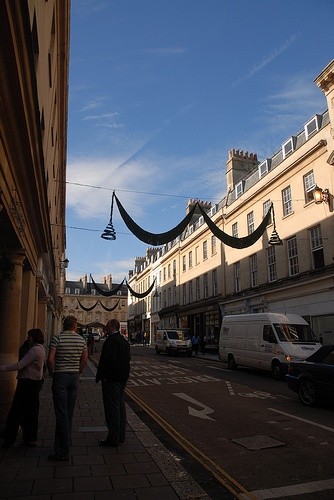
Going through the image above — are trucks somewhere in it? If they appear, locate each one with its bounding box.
[154,330,192,354]
[219,310,323,378]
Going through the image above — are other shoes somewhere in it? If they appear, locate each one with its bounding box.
[118,437,125,443]
[99,440,117,447]
[48,453,69,461]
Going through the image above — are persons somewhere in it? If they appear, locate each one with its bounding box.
[0,328,46,447]
[87,327,94,354]
[192,332,206,355]
[48,316,88,461]
[95,319,131,447]
